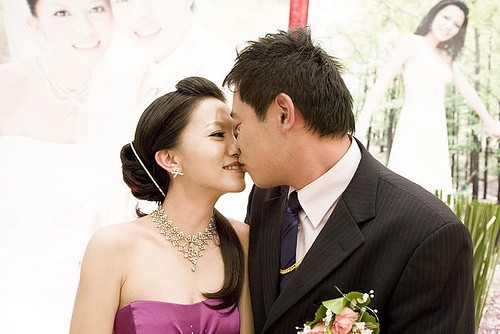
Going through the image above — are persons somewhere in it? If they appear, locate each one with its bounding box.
[69,77,254,334]
[0,0,209,167]
[222,25,475,334]
[353,0,500,191]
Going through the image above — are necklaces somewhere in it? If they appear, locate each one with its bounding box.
[280,259,305,274]
[149,206,216,272]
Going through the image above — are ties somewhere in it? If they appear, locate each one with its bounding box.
[278,190,303,296]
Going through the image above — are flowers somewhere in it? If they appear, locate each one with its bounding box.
[295,285,380,334]
